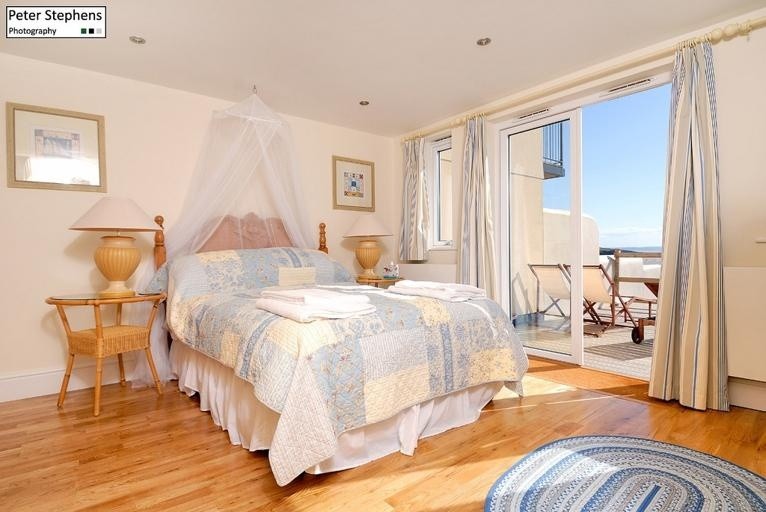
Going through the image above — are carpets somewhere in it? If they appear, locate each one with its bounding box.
[480,427,766,512]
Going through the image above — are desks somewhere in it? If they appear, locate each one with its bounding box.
[42,288,169,417]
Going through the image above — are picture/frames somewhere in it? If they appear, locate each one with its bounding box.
[329,154,376,213]
[2,101,108,194]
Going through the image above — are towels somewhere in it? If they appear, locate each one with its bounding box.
[251,278,488,327]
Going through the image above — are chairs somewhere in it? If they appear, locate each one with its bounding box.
[562,261,641,338]
[610,273,659,345]
[524,259,602,334]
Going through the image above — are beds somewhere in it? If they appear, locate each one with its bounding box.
[161,206,530,491]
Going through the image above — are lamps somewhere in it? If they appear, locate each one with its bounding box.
[339,212,396,281]
[63,195,167,302]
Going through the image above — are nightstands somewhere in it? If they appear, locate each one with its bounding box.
[352,274,405,291]
